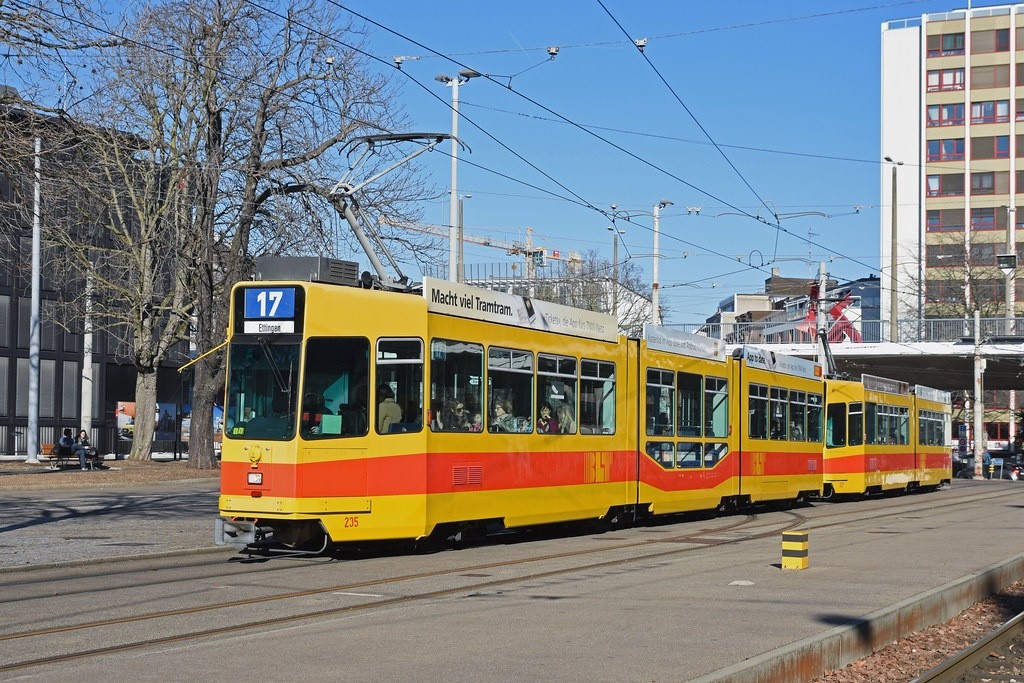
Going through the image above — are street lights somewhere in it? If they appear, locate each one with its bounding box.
[435,68,480,283]
[883,157,904,343]
[447,189,473,285]
[607,227,626,315]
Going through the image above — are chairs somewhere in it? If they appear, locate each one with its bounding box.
[271,387,418,436]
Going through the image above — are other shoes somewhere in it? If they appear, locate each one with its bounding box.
[81,463,110,471]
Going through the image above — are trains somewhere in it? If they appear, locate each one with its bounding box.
[214,281,950,566]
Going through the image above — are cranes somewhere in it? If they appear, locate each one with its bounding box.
[378,217,588,281]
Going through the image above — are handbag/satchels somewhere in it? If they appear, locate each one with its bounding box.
[982,457,987,463]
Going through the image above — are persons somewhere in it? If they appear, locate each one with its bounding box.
[58,428,93,471]
[76,429,98,470]
[982,446,993,480]
[160,409,173,436]
[301,378,948,454]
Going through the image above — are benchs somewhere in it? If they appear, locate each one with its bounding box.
[41,444,94,471]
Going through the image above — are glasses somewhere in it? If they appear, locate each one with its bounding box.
[454,406,465,413]
[495,406,503,410]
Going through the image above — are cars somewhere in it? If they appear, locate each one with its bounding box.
[121,420,135,437]
